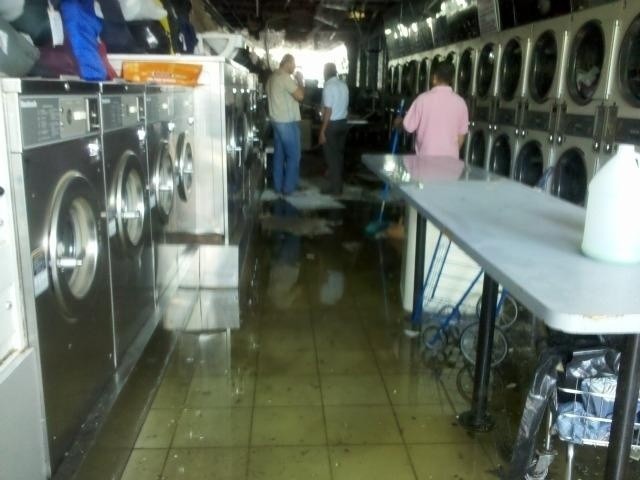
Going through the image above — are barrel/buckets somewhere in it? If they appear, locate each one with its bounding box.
[581,144,640,263]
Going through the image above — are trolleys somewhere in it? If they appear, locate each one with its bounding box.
[422,164,558,368]
[522,313,640,480]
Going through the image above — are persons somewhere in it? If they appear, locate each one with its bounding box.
[314,235,355,322]
[259,223,305,315]
[391,60,469,157]
[266,53,306,195]
[316,62,350,196]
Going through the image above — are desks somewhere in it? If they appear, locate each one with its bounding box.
[359,152,640,479]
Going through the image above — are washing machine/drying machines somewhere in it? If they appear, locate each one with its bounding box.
[0,52,274,479]
[375,0,640,208]
